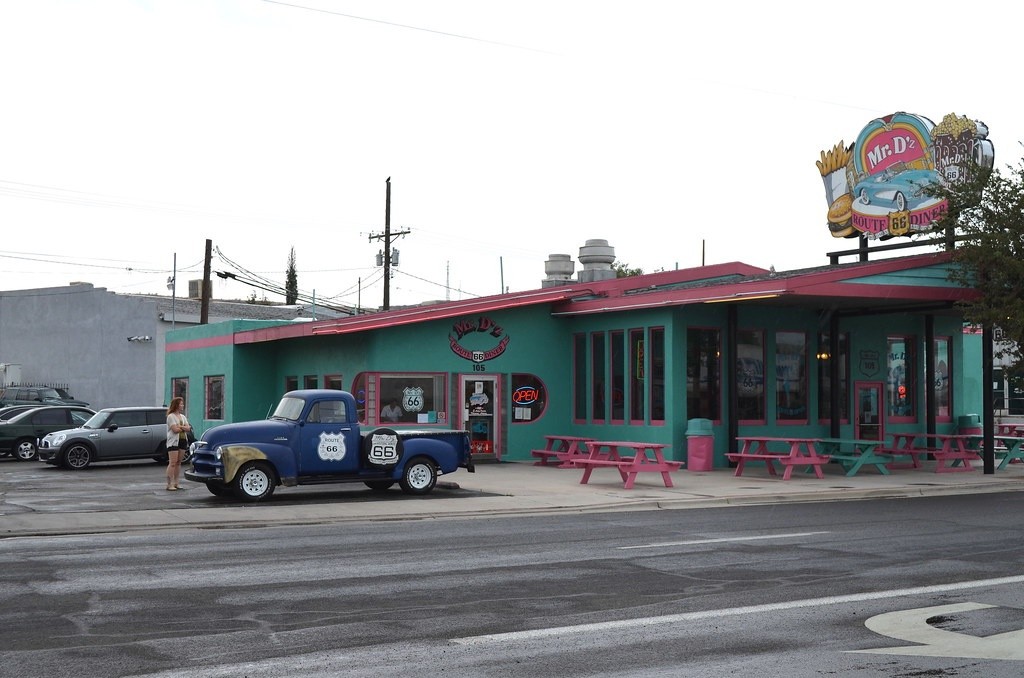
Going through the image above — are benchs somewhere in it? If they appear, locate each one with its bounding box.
[530,450,612,460]
[723,453,832,466]
[953,447,1024,461]
[923,450,982,461]
[874,447,935,455]
[570,456,685,472]
[805,453,895,466]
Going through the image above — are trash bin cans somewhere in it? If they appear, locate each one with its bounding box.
[685,418,715,471]
[955,414,983,448]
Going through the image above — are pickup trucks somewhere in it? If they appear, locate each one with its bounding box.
[184,389,476,502]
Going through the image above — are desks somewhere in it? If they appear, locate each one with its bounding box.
[923,434,983,474]
[994,423,1024,448]
[529,435,612,469]
[723,436,831,481]
[952,434,1024,471]
[570,441,686,489]
[874,434,934,470]
[804,439,894,478]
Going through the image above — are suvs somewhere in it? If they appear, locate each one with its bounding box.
[0,387,90,410]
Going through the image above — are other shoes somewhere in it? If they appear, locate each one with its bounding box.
[166,485,175,491]
[174,483,183,489]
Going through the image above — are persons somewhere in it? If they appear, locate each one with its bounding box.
[381,397,403,423]
[165,397,191,491]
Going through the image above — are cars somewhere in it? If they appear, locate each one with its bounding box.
[38,407,196,470]
[0,405,45,420]
[0,407,98,462]
